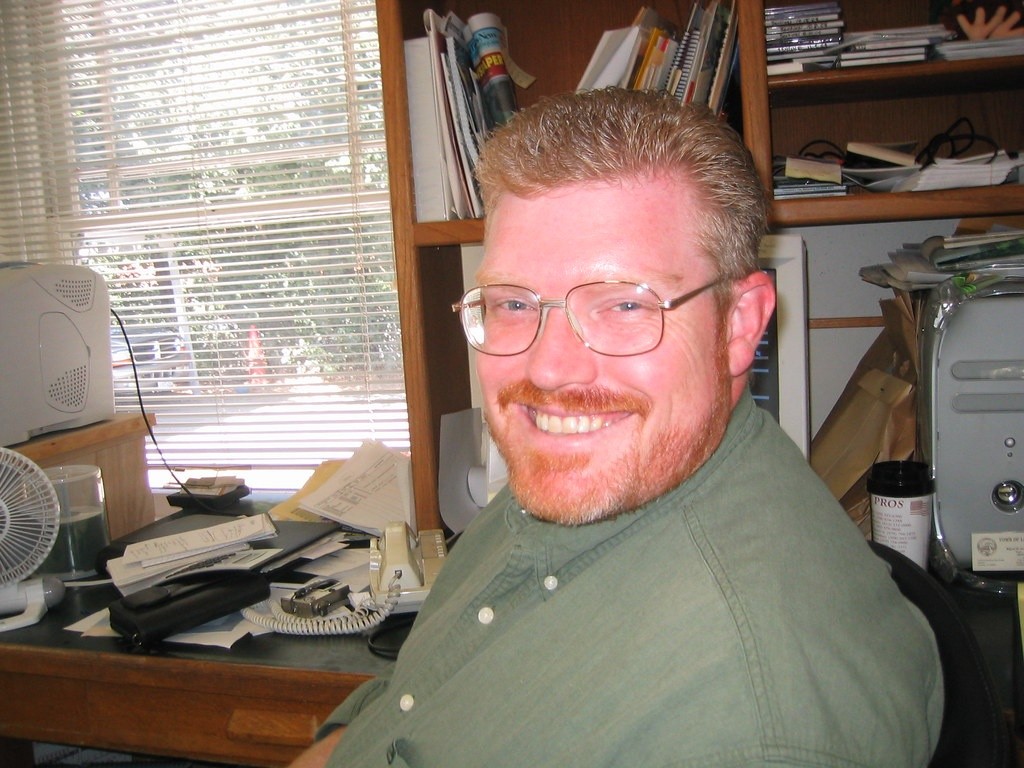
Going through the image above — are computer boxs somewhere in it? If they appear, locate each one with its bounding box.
[919,285,1024,594]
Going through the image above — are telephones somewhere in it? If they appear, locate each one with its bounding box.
[367,520,450,613]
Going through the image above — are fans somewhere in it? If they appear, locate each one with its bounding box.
[0,447,61,633]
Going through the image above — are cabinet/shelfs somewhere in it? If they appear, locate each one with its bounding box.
[373,0,1022,583]
[8,410,158,546]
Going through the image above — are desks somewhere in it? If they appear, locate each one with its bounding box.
[0,605,397,768]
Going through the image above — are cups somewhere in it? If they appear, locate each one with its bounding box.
[23,463,110,582]
[868,460,933,568]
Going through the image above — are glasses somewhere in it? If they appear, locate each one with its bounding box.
[451,280,720,357]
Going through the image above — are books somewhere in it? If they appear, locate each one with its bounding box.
[764,4,1024,76]
[403,9,487,222]
[575,0,739,118]
[774,138,1024,201]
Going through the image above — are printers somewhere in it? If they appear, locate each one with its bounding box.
[0,262,114,445]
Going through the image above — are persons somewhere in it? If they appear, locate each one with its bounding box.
[286,85,944,768]
